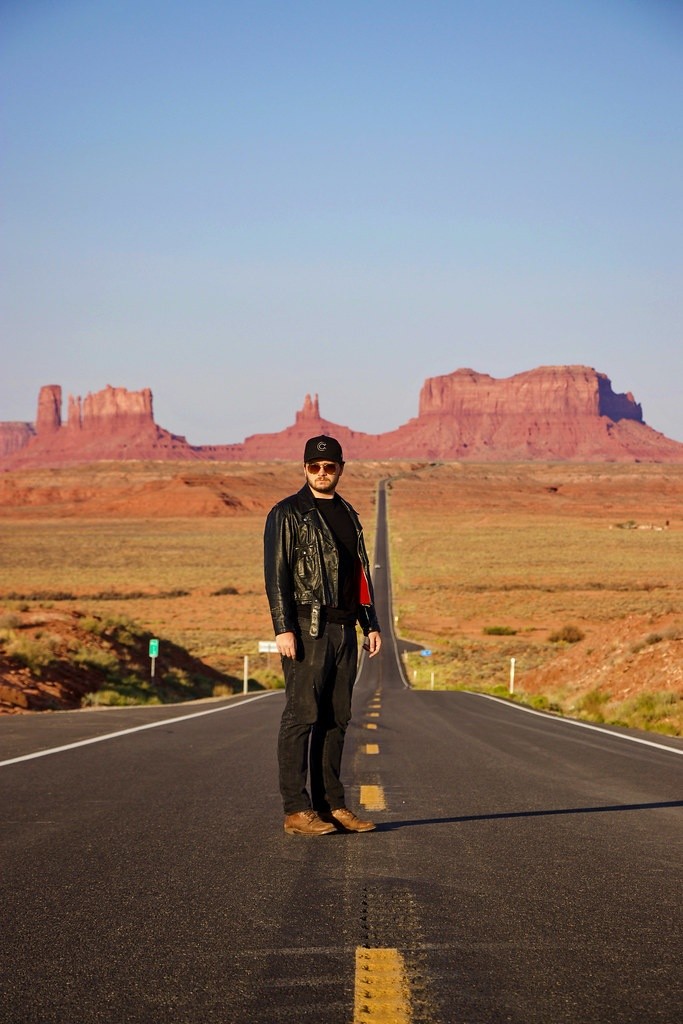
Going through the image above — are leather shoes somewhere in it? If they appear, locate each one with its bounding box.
[284,808,337,835]
[331,809,376,832]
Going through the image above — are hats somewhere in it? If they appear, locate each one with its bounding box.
[304,435,343,464]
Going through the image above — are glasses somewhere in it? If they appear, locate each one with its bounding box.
[307,462,342,475]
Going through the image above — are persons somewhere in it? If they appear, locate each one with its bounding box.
[263,434,382,836]
